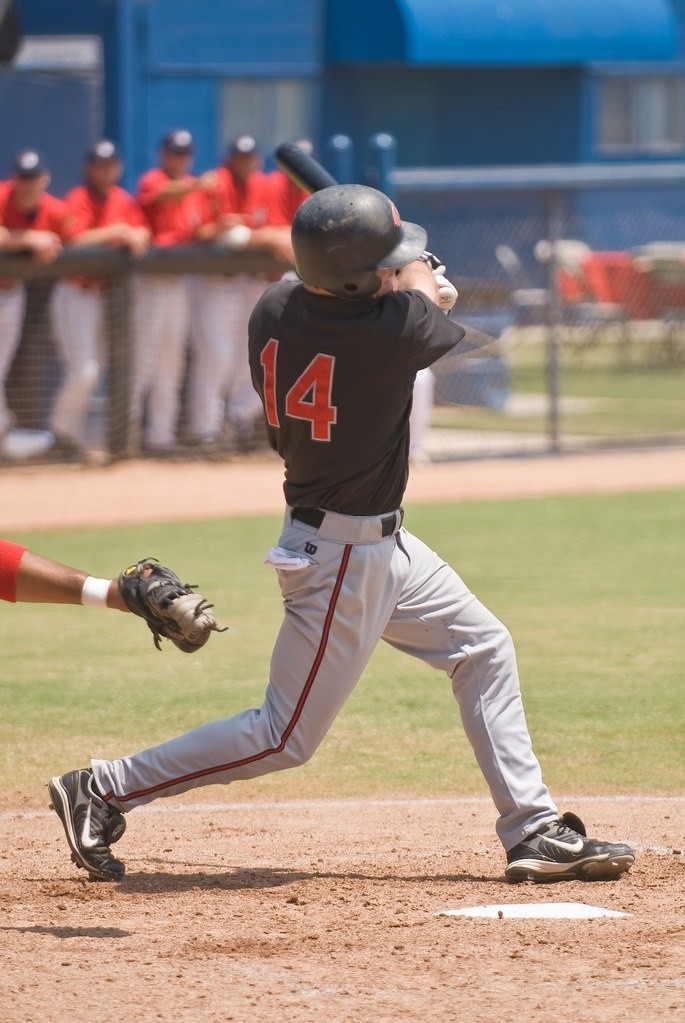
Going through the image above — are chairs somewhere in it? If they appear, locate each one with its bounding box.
[493,239,685,367]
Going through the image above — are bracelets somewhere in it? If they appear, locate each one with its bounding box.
[80,575,113,608]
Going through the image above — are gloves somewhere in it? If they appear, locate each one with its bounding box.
[420,250,458,317]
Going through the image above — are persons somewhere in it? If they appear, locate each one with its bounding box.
[49,139,152,462]
[130,130,217,454]
[550,235,685,320]
[0,537,228,653]
[48,186,633,882]
[0,149,72,460]
[183,135,319,457]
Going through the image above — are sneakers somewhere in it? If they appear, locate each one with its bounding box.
[44,767,127,883]
[505,811,635,885]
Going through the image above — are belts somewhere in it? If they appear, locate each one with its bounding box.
[290,505,404,538]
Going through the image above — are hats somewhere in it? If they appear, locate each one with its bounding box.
[9,145,49,178]
[228,133,261,156]
[161,128,195,153]
[82,138,119,164]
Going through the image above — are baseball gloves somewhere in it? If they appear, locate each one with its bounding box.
[118,556,230,654]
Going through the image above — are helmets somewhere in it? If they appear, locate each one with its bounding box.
[291,183,428,299]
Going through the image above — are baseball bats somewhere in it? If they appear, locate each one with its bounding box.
[272,140,458,312]
